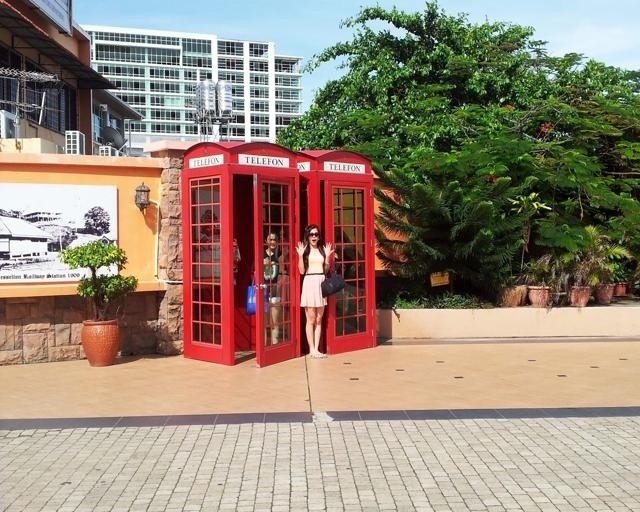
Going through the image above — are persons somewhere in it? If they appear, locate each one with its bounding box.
[263,232,289,346]
[194,210,241,286]
[296,223,334,359]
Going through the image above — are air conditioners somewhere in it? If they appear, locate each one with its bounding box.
[99,146,116,156]
[65,131,86,155]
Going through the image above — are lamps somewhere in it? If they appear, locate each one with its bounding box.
[134,182,151,212]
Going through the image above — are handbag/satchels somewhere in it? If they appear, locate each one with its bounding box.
[321,275,345,297]
[246,286,269,314]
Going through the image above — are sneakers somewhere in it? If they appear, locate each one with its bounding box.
[309,353,327,358]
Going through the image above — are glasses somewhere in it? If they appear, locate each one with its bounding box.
[310,233,319,236]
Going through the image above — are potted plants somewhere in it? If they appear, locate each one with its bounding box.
[59,239,138,367]
[500,194,640,307]
